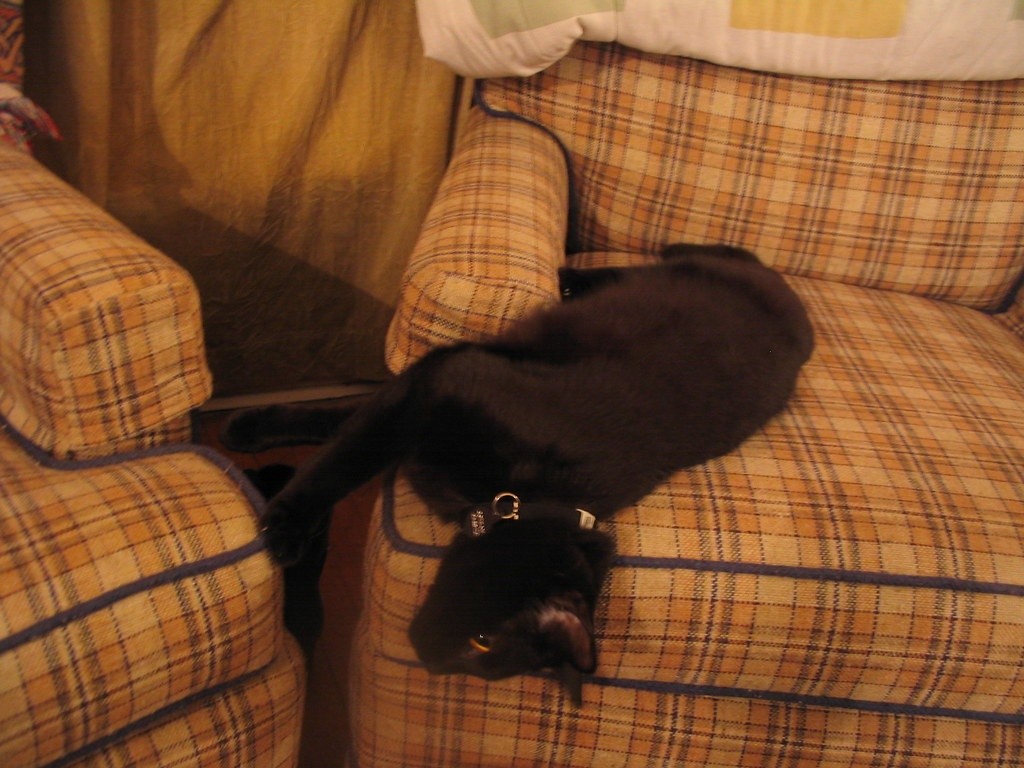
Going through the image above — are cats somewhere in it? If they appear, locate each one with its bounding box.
[262,243,816,711]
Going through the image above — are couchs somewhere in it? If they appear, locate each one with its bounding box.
[347,2,1024,768]
[0,1,308,768]
